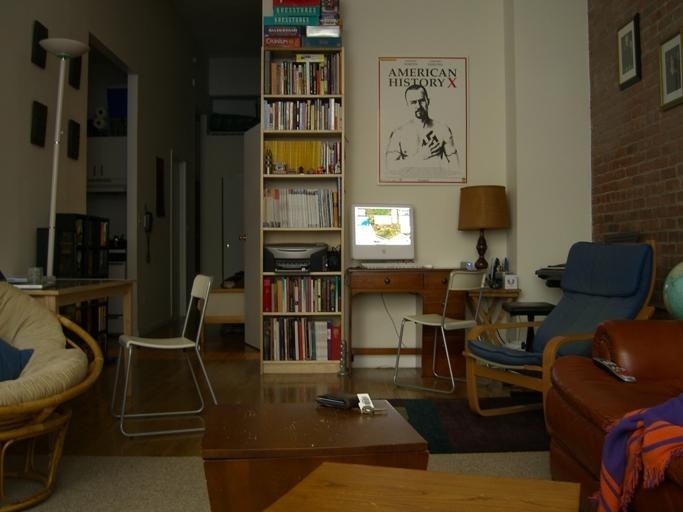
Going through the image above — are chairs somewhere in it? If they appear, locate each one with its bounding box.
[459,236,655,429]
[113,272,225,446]
[0,274,108,512]
[390,265,497,397]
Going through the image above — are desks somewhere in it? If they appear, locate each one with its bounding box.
[344,266,470,383]
[193,395,432,512]
[199,266,245,350]
[468,287,520,350]
[9,276,137,358]
[260,459,585,512]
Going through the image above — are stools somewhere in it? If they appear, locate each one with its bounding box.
[501,301,558,354]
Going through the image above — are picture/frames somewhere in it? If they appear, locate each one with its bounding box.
[613,12,644,92]
[654,28,682,114]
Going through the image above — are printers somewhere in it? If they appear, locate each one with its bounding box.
[263,243,328,272]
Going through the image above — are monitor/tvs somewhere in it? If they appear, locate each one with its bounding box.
[350,202,414,261]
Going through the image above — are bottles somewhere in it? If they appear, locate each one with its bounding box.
[491,257,501,281]
[502,257,509,274]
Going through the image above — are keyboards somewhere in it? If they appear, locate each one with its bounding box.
[361,263,418,267]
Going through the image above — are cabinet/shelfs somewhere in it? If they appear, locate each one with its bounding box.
[87,135,126,195]
[48,210,109,365]
[253,44,351,379]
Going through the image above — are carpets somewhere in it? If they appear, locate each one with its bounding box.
[374,386,551,455]
[0,453,555,512]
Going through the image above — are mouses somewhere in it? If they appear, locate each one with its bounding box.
[423,265,432,268]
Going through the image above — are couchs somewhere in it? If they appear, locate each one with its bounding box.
[538,309,682,512]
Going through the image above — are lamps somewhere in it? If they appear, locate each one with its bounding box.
[455,184,513,270]
[35,33,89,286]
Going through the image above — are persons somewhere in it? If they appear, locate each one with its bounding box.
[383,84,462,171]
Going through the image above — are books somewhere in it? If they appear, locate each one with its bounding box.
[60,212,112,374]
[11,282,48,290]
[261,0,344,365]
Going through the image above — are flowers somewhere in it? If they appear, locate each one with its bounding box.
[86,104,107,134]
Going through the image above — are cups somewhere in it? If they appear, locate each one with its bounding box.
[26,266,44,285]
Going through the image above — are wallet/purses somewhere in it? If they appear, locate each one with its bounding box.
[314,392,360,411]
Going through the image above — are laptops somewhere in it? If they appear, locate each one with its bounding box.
[0,268,50,290]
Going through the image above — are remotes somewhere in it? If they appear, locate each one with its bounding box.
[593,356,638,384]
